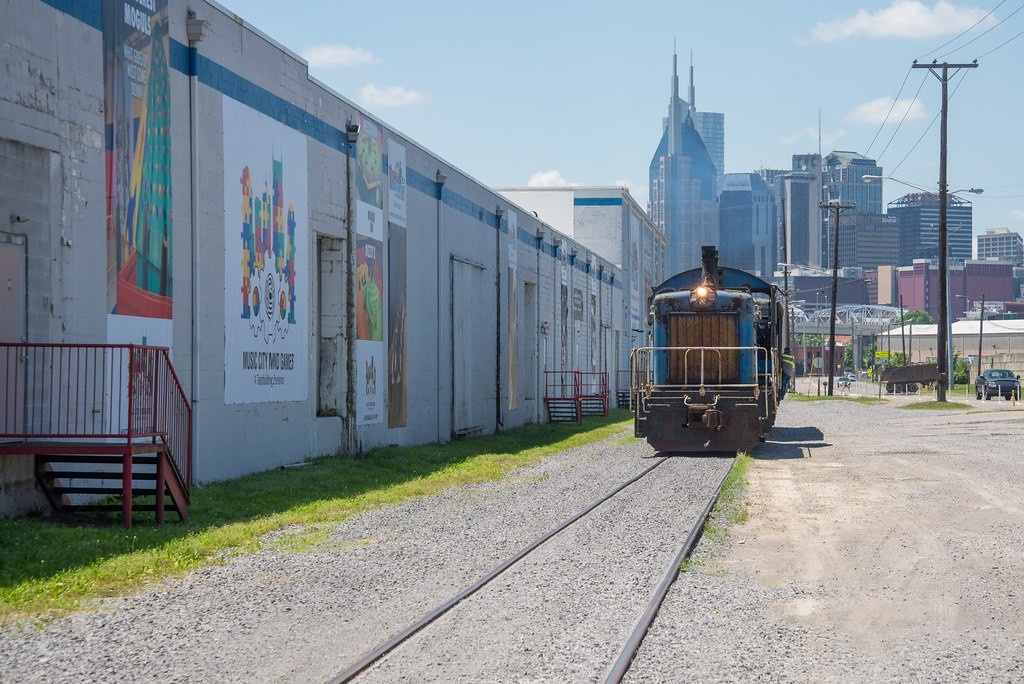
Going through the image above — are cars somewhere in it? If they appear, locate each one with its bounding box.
[974,369,1022,401]
[836,377,851,388]
[847,374,856,381]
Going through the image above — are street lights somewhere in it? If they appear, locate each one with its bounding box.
[777,262,838,396]
[954,294,989,378]
[861,174,984,392]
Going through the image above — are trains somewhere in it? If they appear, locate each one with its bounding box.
[629,245,793,454]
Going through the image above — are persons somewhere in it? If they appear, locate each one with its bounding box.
[781,347,795,400]
